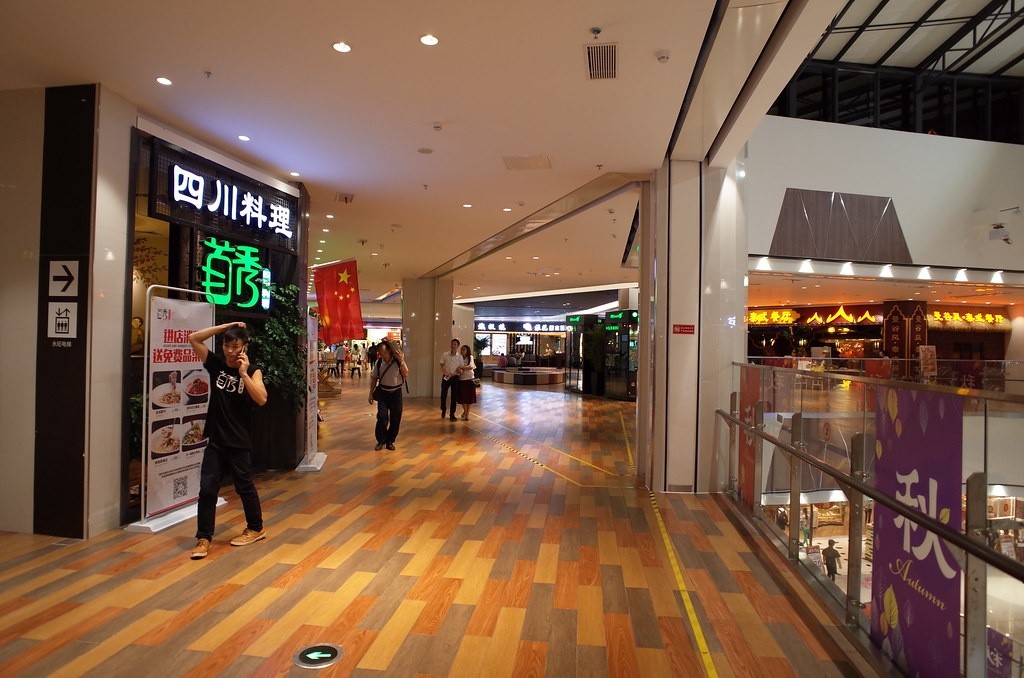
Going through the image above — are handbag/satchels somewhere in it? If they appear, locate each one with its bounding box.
[357,358,361,365]
[372,385,382,402]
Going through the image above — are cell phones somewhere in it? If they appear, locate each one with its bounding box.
[241,345,248,355]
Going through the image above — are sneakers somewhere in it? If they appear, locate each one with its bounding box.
[230,527,267,546]
[375,442,385,450]
[449,414,458,420]
[190,536,212,559]
[471,377,481,389]
[441,409,446,418]
[386,443,395,450]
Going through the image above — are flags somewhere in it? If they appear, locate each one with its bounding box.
[313,256,366,347]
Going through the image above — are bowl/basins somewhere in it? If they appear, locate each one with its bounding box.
[183,373,208,403]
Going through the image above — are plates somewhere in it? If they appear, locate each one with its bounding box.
[182,420,207,445]
[153,382,181,407]
[151,423,180,453]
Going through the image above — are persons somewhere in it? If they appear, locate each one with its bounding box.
[367,340,409,452]
[455,344,478,421]
[799,507,810,548]
[439,338,464,421]
[324,339,376,381]
[508,341,565,367]
[497,353,508,367]
[788,336,895,381]
[187,320,269,559]
[864,500,873,526]
[774,505,788,532]
[821,539,843,582]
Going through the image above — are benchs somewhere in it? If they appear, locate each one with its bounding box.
[481,366,567,386]
